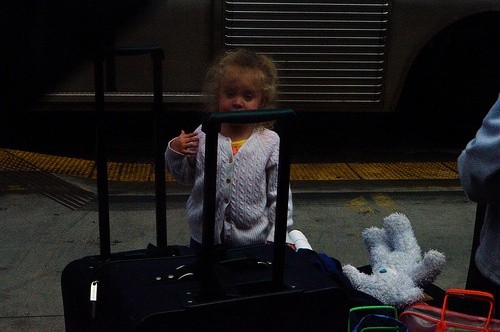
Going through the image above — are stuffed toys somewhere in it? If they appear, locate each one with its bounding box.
[341,211,447,310]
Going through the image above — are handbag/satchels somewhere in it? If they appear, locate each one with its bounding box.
[400,288,500,331]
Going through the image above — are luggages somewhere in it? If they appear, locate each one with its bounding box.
[61,45,194,331]
[83,108,345,331]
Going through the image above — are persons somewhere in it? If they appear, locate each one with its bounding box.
[457,93,499,319]
[164,48,297,250]
